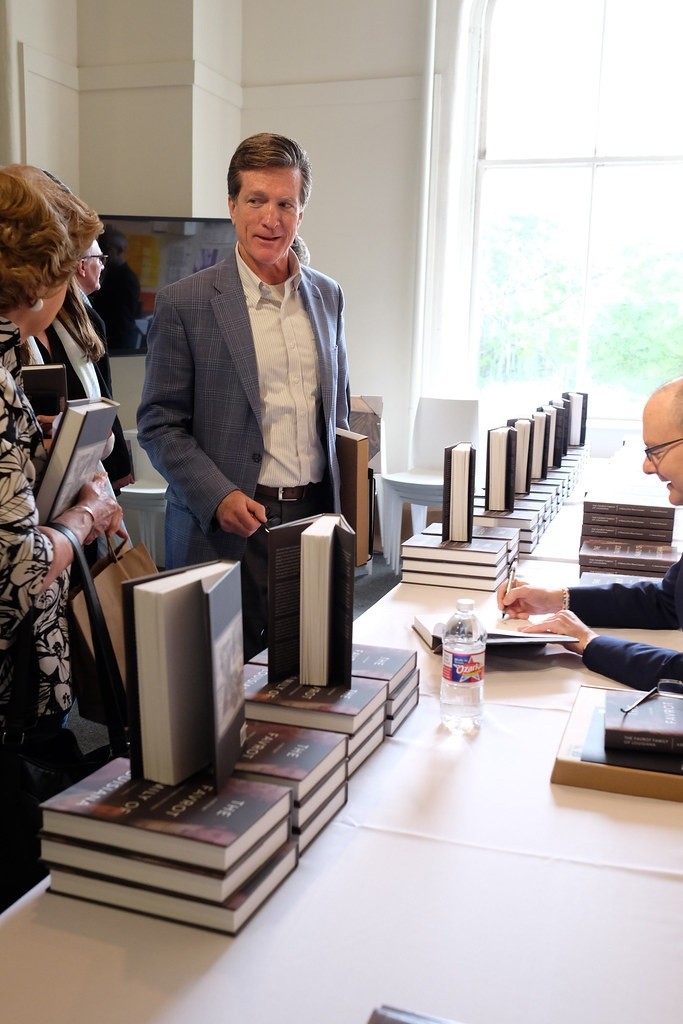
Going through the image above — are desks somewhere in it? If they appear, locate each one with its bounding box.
[2,459,683,1023]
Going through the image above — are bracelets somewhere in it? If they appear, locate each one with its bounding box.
[64,506,95,524]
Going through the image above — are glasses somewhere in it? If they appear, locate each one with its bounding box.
[78,254,108,266]
[644,438,683,462]
[620,678,683,713]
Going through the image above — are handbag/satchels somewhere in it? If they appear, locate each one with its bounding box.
[0,523,128,915]
[70,530,160,693]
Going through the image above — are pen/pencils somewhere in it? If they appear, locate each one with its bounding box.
[501,567,515,619]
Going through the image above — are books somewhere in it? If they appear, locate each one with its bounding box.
[335,428,376,567]
[39,641,417,937]
[33,397,120,526]
[579,476,677,586]
[400,393,587,591]
[266,514,355,690]
[21,364,67,421]
[550,685,683,802]
[412,606,580,656]
[369,1004,464,1023]
[121,560,245,788]
[125,440,135,481]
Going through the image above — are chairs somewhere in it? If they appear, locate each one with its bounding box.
[116,431,168,564]
[375,398,478,573]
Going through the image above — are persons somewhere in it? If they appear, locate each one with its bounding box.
[0,164,146,858]
[136,133,352,663]
[92,230,141,350]
[497,375,683,692]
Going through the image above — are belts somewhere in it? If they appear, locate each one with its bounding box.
[256,483,309,501]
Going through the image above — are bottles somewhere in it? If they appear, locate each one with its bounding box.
[440,600,487,733]
